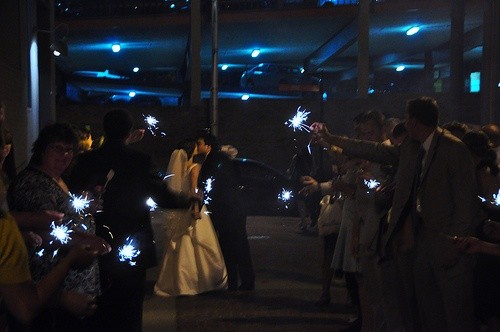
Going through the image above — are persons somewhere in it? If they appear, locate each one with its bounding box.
[0,130,100,331]
[152,141,233,298]
[7,123,104,331]
[193,134,256,297]
[70,109,205,332]
[290,94,500,332]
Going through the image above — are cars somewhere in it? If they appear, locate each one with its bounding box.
[239,62,325,100]
[229,156,297,217]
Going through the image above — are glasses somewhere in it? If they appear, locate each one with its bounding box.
[44,142,75,155]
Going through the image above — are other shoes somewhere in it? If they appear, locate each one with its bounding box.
[346,314,363,332]
[241,272,255,293]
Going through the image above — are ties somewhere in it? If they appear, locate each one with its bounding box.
[411,142,424,216]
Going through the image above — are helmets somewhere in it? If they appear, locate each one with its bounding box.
[461,130,491,151]
[480,124,500,137]
